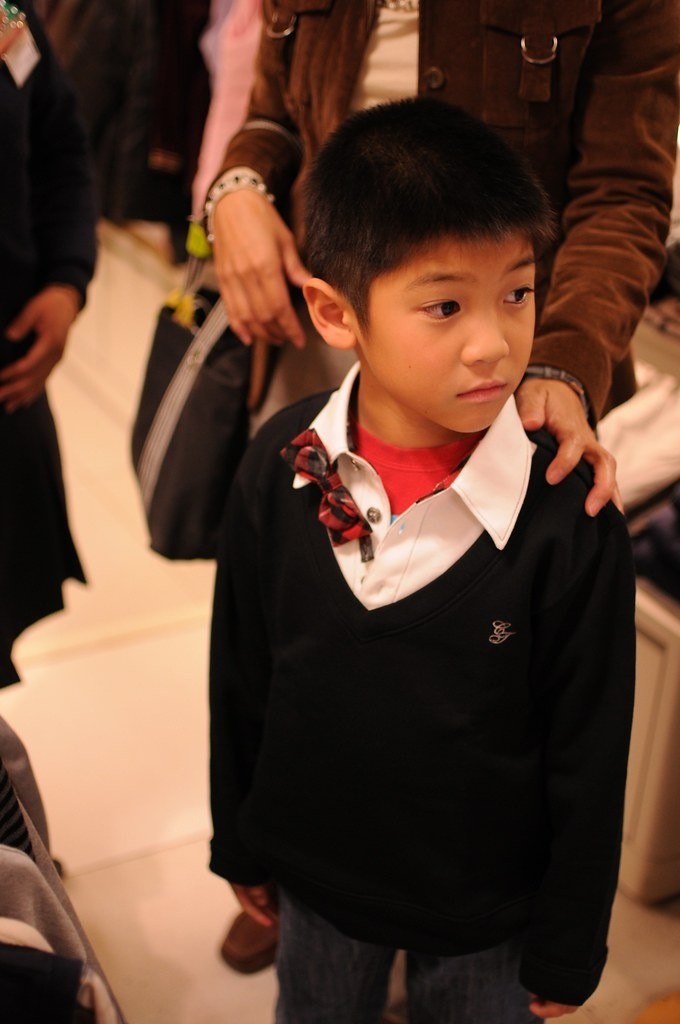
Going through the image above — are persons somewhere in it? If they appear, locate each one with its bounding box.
[202,0,680,976]
[209,97,636,1024]
[0,0,98,691]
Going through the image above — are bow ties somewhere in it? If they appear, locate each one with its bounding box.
[279,428,373,562]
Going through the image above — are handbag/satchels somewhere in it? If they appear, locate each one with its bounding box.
[131,120,307,560]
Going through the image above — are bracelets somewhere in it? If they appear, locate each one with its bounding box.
[528,369,590,416]
[204,175,275,243]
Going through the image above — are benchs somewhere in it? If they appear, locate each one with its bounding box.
[66,220,680,906]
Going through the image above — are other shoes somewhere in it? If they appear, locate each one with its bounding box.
[220,891,280,973]
